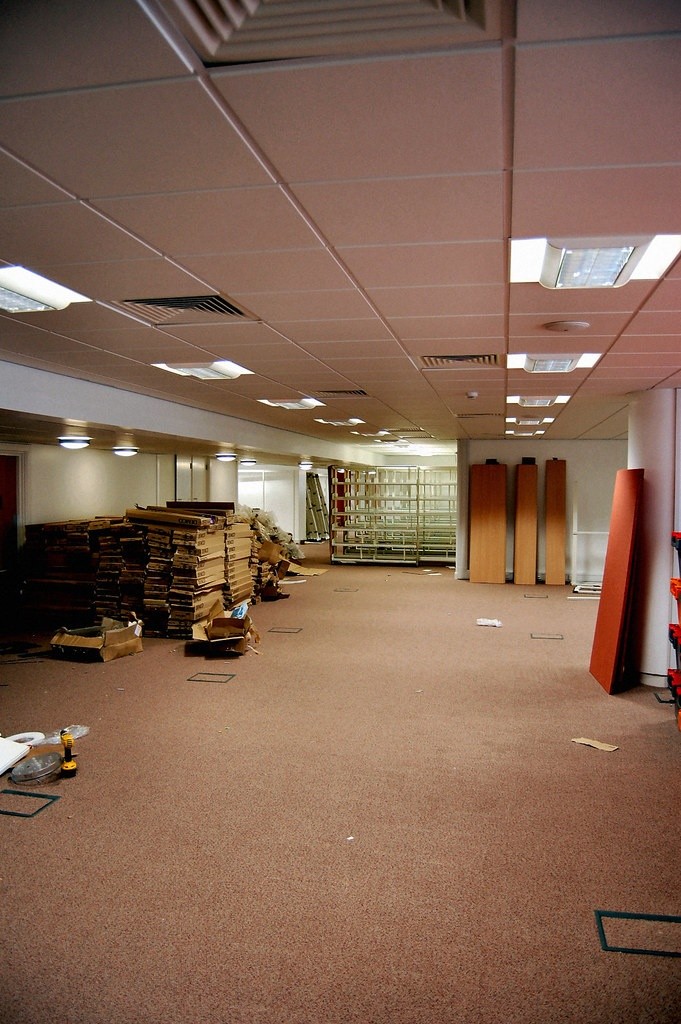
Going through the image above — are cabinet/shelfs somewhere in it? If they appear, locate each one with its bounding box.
[328,465,457,567]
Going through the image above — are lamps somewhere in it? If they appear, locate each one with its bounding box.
[57,436,314,471]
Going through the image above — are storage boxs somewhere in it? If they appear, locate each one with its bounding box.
[20,501,329,663]
